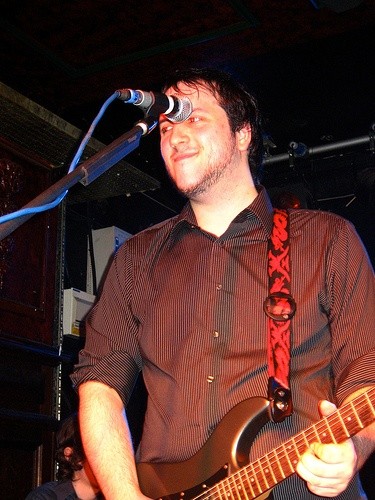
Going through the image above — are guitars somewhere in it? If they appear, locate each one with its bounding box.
[133,383,375,499]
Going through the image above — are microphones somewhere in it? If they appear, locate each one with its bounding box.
[115,88,193,125]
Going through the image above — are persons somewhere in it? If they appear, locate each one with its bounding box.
[24,411,107,500]
[69,64,375,499]
